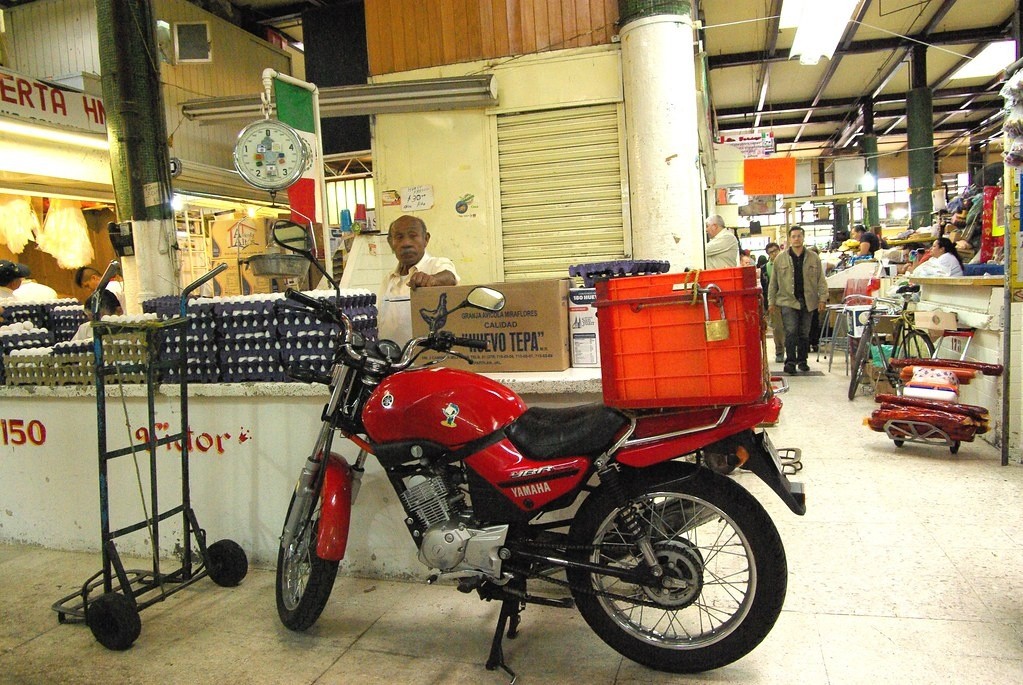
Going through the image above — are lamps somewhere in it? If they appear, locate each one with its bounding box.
[181,72,498,116]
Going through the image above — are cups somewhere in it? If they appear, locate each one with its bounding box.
[354,203,366,221]
[341,209,352,231]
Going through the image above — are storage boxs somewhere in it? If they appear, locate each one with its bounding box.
[409,280,568,374]
[206,259,272,298]
[596,263,765,408]
[208,217,267,258]
[847,304,889,384]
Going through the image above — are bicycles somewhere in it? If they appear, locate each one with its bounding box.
[842,283,936,400]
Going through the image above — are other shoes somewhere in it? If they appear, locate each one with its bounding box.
[776,355,784,362]
[798,363,810,371]
[784,364,796,374]
[812,344,818,352]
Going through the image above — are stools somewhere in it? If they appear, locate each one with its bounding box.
[816,278,881,376]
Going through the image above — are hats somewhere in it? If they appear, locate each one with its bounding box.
[0,260,31,286]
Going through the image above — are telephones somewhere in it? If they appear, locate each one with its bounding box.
[108,221,134,256]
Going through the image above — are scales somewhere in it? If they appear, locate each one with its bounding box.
[231,116,319,278]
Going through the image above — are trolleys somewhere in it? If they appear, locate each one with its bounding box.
[50,261,249,649]
[883,325,976,453]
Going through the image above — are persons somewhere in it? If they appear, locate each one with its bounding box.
[705,215,964,374]
[377,215,461,351]
[70,266,123,340]
[0,259,57,302]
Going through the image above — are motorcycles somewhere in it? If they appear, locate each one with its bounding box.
[272,219,806,685]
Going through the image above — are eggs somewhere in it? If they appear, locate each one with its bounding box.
[0,288,371,377]
